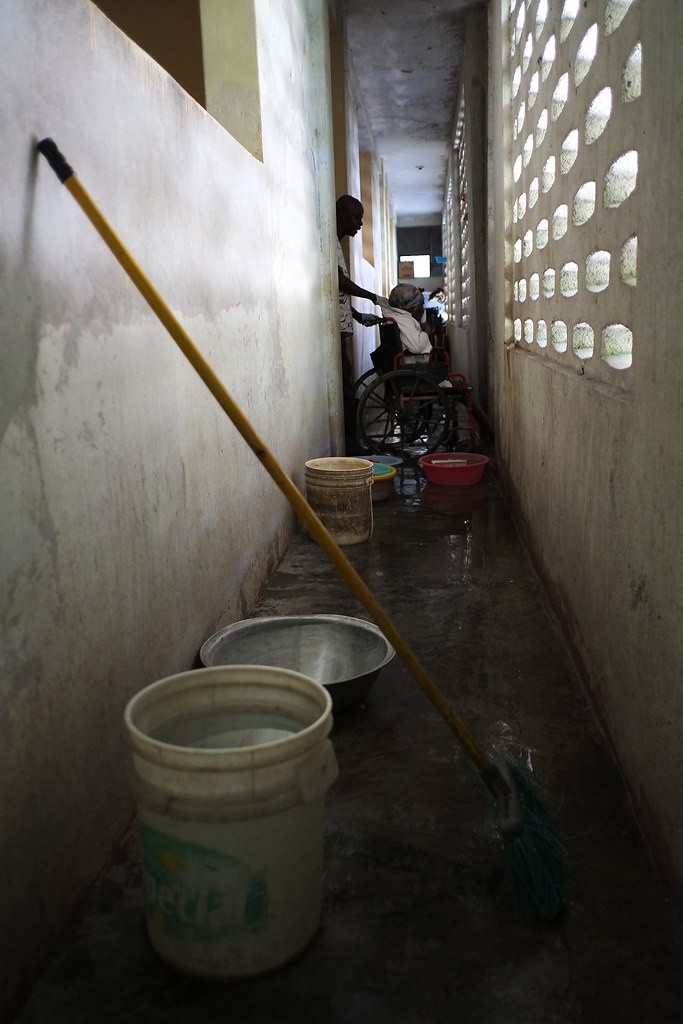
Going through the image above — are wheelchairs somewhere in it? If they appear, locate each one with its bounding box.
[351,318,478,463]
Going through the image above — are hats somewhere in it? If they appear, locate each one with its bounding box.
[389,284,423,310]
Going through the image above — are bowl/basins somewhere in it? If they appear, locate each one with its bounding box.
[199,614,398,710]
[354,455,404,468]
[372,463,397,502]
[417,452,489,487]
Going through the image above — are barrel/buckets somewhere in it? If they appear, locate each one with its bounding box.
[122,663,340,979]
[304,458,375,546]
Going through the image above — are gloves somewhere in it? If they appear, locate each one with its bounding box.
[373,294,389,308]
[361,313,378,327]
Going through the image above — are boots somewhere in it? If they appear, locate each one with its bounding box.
[344,399,372,456]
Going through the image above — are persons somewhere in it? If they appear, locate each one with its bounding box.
[381,283,495,445]
[336,194,391,457]
